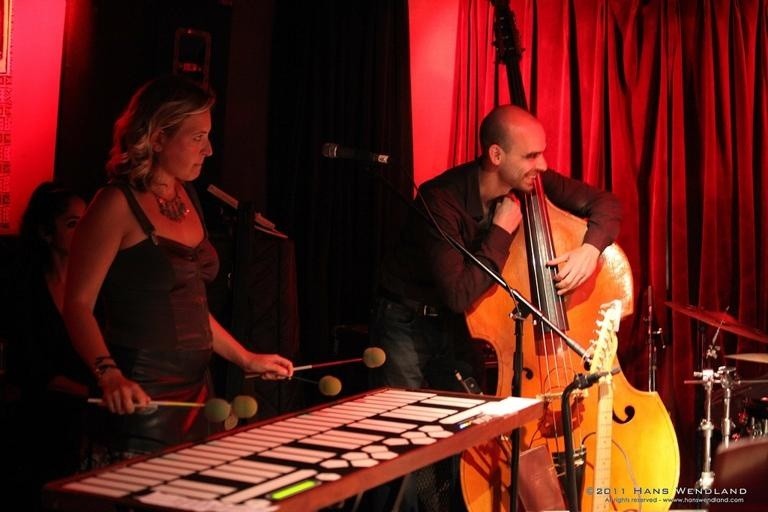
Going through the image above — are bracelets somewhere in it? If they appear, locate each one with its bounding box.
[93,356,119,384]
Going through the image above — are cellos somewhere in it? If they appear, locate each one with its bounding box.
[459,0,681,512]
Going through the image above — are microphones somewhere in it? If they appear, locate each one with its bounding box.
[321,142,394,165]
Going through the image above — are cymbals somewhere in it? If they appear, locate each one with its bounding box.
[724,354,768,364]
[663,300,767,343]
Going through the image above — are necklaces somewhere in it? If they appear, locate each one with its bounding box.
[148,187,186,222]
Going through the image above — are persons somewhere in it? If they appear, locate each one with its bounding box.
[18,180,86,318]
[409,105,622,512]
[62,76,295,456]
